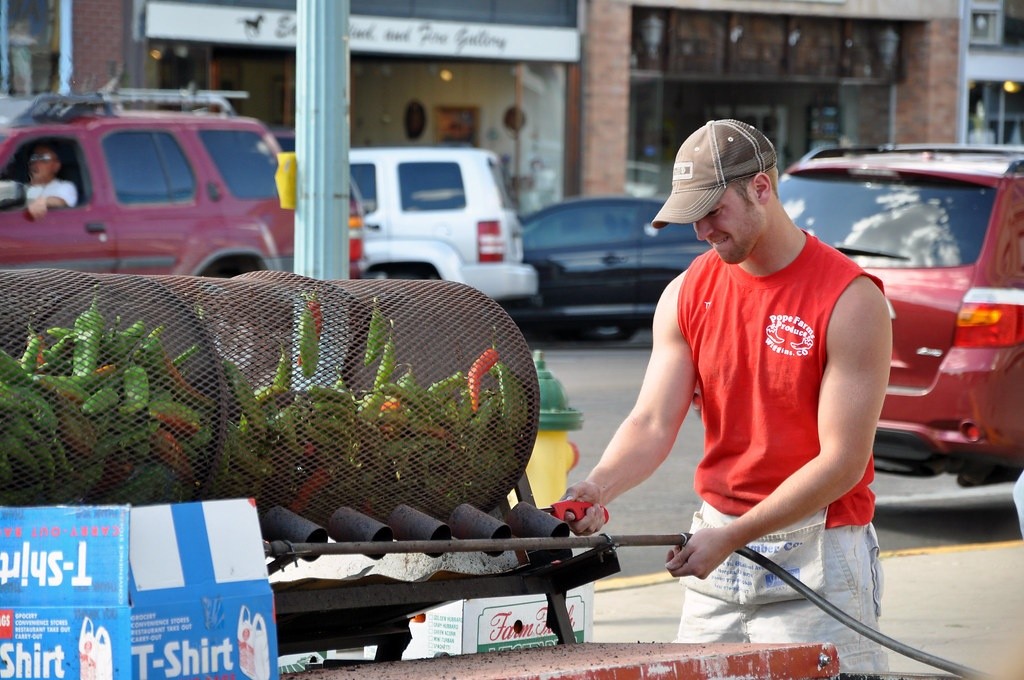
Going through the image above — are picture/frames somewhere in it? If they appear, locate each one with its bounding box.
[431,103,480,147]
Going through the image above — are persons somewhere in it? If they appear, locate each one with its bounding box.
[14,148,78,220]
[552,119,894,673]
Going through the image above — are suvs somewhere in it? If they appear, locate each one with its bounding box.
[776,144,1024,488]
[2,80,295,279]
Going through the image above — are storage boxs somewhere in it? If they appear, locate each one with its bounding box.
[361,581,593,660]
[0,499,279,680]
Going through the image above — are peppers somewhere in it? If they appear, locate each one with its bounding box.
[0,284,531,519]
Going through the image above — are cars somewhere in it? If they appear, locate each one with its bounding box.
[348,145,538,301]
[275,132,362,280]
[499,197,713,342]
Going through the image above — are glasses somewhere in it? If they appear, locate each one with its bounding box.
[28,151,53,163]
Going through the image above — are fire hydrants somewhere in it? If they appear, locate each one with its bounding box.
[507,350,585,511]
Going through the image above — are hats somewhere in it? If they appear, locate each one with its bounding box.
[651,118,778,228]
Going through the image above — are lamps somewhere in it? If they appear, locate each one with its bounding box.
[877,29,898,69]
[150,43,167,58]
[437,60,455,82]
[640,12,664,64]
